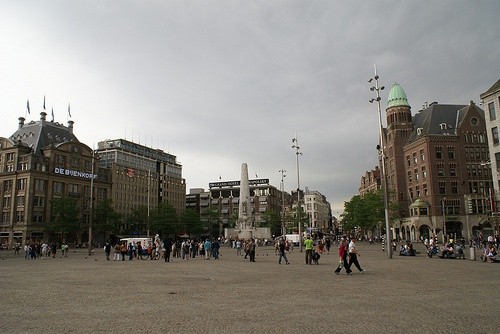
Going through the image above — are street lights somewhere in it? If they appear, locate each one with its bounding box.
[367,63,392,258]
[291,131,303,253]
[277,168,286,239]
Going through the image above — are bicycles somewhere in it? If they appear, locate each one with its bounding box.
[139,246,160,260]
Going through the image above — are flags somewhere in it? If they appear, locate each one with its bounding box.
[43,95,45,109]
[27,100,30,114]
[68,102,71,117]
[52,107,54,121]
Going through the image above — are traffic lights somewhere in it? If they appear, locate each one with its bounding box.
[467,198,473,214]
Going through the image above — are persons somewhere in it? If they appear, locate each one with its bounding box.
[103,236,220,263]
[335,236,352,275]
[221,233,500,264]
[5,240,69,260]
[304,235,314,265]
[279,241,290,265]
[348,237,367,273]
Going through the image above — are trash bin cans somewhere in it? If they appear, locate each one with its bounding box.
[470,247,476,261]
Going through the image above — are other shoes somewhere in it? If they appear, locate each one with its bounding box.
[360,270,366,273]
[286,262,290,264]
[244,258,248,259]
[279,262,281,264]
[346,272,352,275]
[249,260,256,262]
[335,271,339,275]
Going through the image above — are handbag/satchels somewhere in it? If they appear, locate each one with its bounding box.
[284,249,286,254]
[339,260,344,268]
